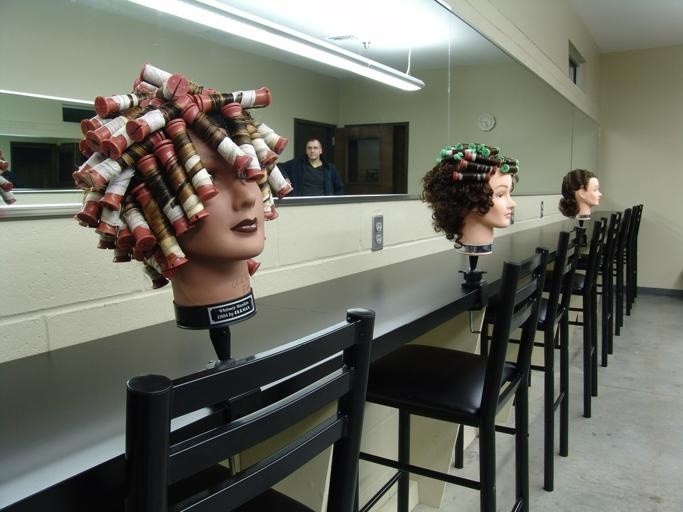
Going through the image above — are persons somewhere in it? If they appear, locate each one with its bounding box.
[422,140,518,254]
[559,169,602,219]
[74,61,293,333]
[286,134,346,195]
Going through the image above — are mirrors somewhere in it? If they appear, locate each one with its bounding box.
[2,2,601,209]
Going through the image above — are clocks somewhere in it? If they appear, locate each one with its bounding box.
[473,109,500,133]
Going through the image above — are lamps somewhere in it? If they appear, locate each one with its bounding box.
[127,2,425,97]
